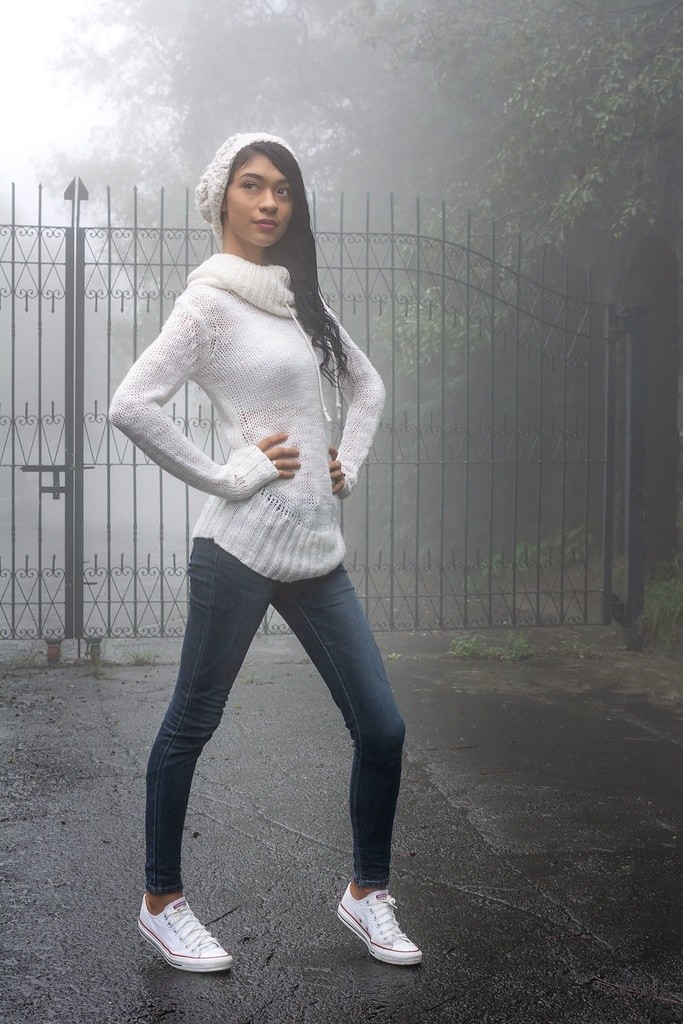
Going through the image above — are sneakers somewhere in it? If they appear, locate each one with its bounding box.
[339,882,422,966]
[138,894,233,972]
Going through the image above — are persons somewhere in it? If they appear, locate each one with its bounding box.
[108,132,424,973]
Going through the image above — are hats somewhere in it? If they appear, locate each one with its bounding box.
[196,132,303,240]
